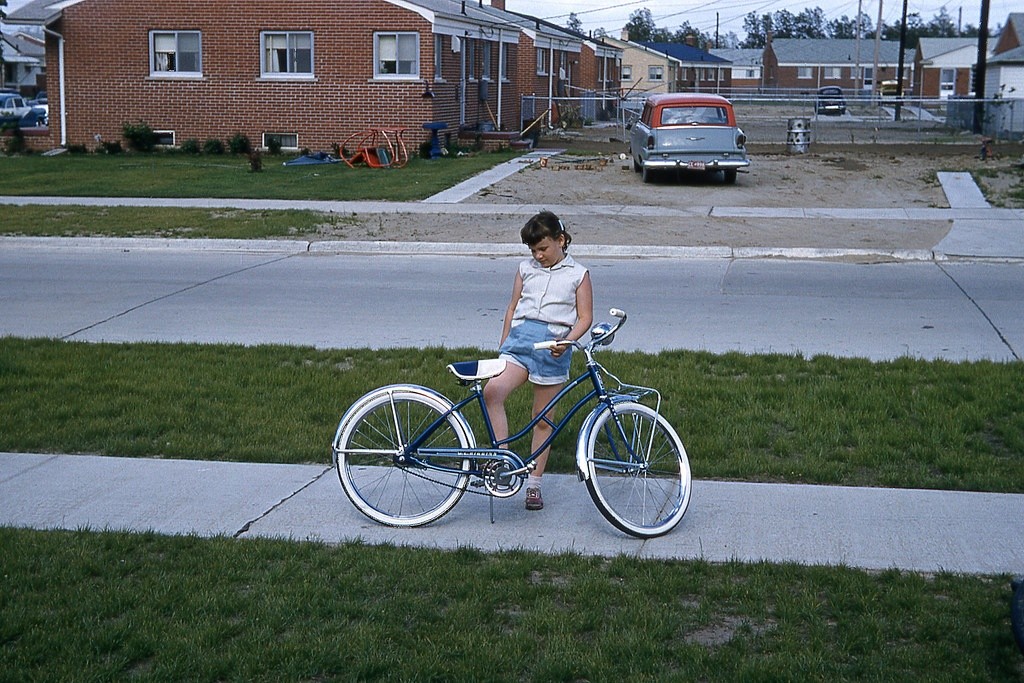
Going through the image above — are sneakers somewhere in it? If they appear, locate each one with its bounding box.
[525,488,542,511]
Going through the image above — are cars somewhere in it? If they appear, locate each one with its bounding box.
[814,85,846,116]
[878,81,904,107]
[629,92,750,185]
[0,87,48,126]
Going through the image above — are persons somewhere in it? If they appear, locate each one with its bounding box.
[483,210,594,509]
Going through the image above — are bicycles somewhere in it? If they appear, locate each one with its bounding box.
[331,309,692,538]
